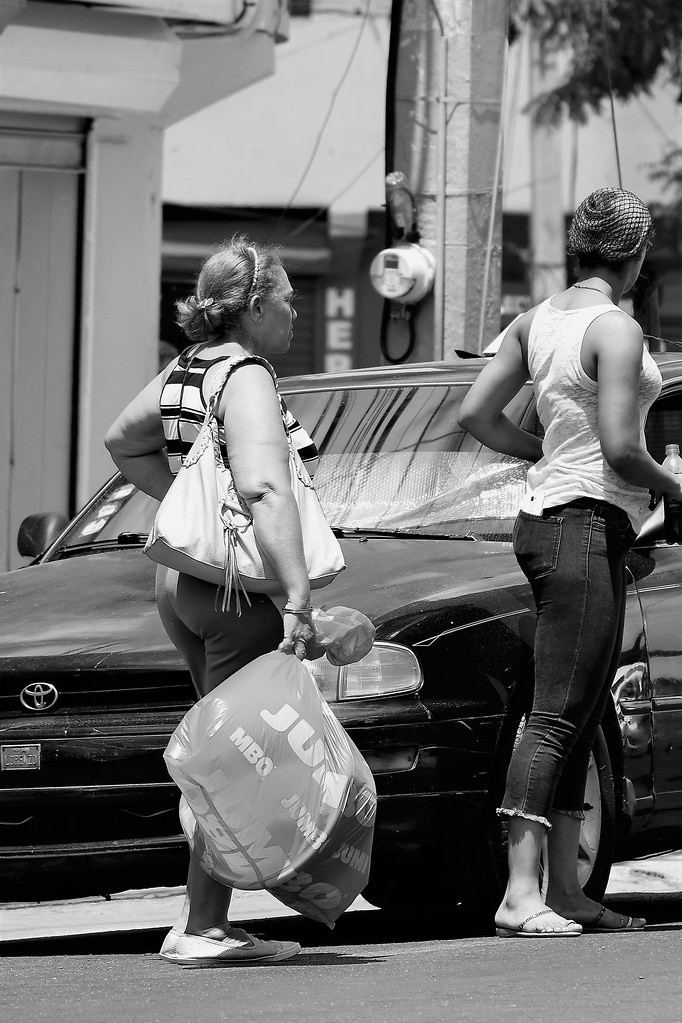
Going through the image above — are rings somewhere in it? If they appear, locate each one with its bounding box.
[298,637,305,644]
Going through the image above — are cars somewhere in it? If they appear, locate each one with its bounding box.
[0,352,681,934]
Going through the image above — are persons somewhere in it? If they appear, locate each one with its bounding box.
[104,245,316,968]
[457,188,681,938]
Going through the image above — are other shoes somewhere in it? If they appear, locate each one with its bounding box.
[158,927,302,966]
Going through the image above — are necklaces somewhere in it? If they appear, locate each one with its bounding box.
[573,284,609,296]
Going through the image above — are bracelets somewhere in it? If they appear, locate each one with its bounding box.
[282,605,314,613]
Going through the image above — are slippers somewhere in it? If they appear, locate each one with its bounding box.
[496,908,581,936]
[578,905,644,932]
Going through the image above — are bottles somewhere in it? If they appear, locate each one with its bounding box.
[386,170,414,235]
[662,444,682,546]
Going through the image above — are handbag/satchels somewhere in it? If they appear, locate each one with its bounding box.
[142,356,346,617]
